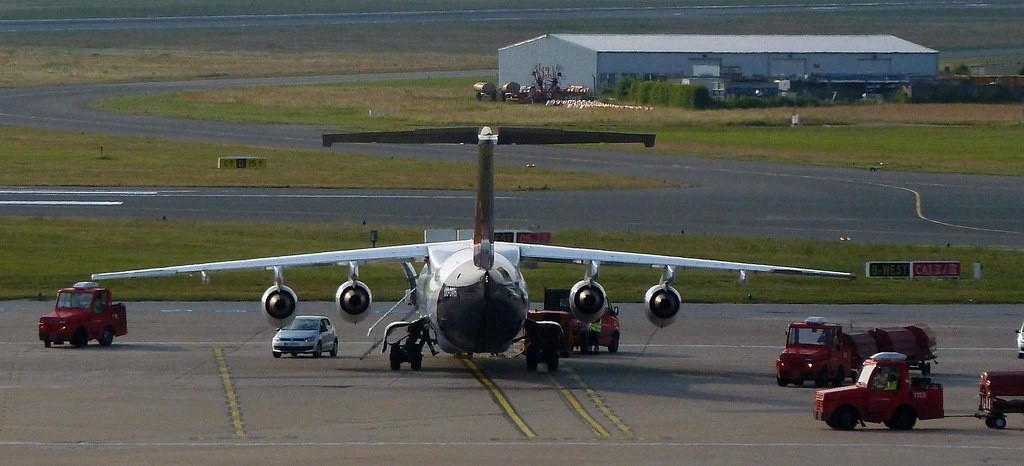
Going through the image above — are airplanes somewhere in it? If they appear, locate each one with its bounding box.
[91,122,856,373]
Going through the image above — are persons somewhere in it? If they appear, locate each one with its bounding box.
[873,364,897,390]
[585,316,603,355]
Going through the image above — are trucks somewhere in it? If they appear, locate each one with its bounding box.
[974,369,1024,429]
[776,316,861,389]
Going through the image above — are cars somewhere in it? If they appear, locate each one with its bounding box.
[1015,322,1024,359]
[271,315,339,359]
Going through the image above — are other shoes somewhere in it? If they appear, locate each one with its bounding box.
[431,350,439,356]
[587,351,592,354]
[594,351,598,355]
[422,355,424,357]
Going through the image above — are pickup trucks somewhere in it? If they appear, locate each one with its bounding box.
[39,281,128,348]
[813,351,945,431]
[527,287,621,354]
